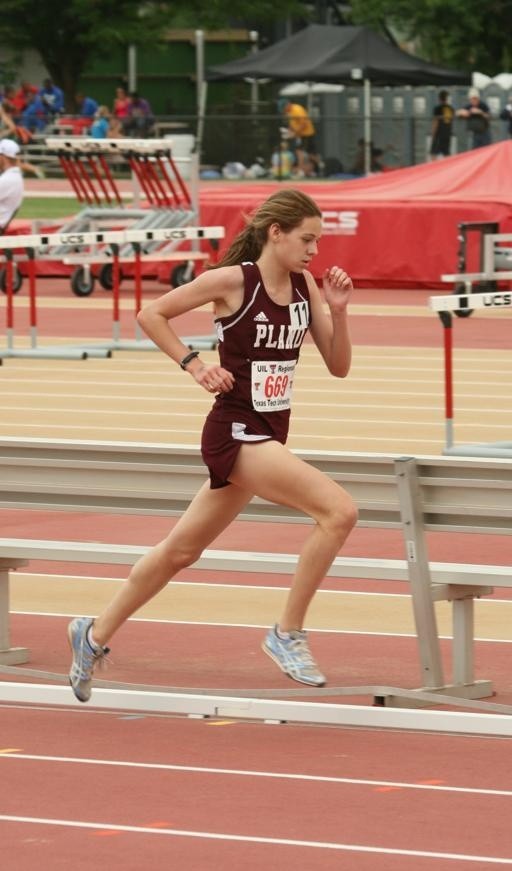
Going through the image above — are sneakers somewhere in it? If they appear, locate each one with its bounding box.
[66,617,108,701]
[262,625,326,688]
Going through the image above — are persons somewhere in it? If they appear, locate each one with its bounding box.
[66,187,358,702]
[454,88,491,149]
[222,98,385,181]
[499,94,512,138]
[0,139,25,235]
[429,90,455,161]
[0,76,154,180]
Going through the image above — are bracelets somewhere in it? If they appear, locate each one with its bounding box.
[179,351,200,370]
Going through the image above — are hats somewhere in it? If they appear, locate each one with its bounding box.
[0,137,21,159]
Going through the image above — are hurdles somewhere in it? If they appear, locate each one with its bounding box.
[430,292,512,458]
